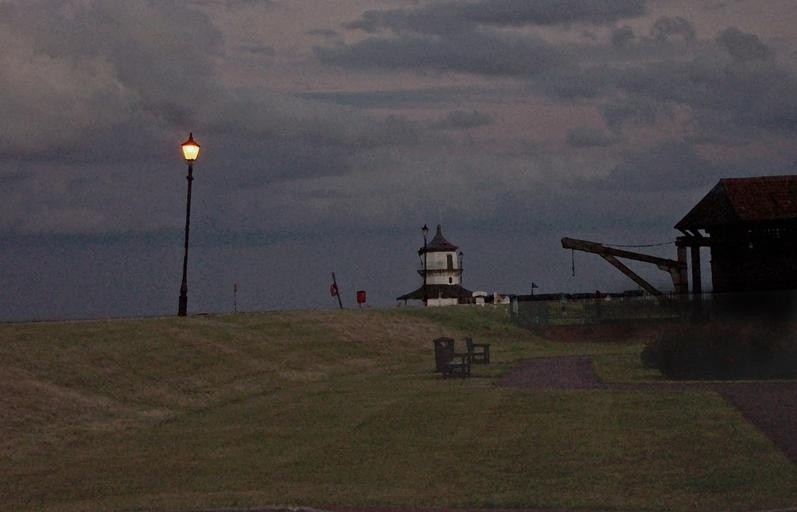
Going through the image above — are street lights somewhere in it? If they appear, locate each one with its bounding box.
[178,134,201,317]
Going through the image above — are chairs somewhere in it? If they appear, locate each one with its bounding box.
[434,333,490,379]
